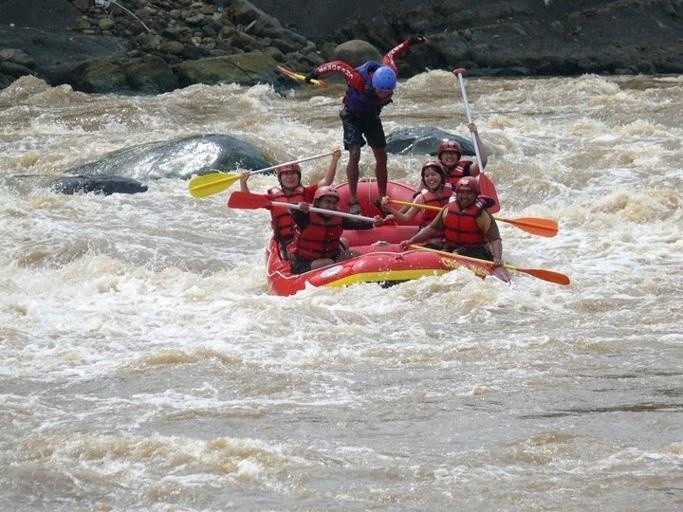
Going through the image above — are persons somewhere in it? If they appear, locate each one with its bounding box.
[376,155,456,228]
[301,33,429,220]
[381,121,489,221]
[236,149,349,257]
[284,185,385,276]
[397,175,503,270]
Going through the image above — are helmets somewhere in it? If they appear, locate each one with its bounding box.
[456,175,480,193]
[278,164,301,181]
[313,186,339,207]
[438,141,461,158]
[421,160,446,175]
[372,67,396,92]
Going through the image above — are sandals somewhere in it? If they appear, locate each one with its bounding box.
[349,199,361,214]
[375,199,391,216]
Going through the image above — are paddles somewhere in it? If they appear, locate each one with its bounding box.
[402,242,571,286]
[453,68,501,213]
[278,65,329,87]
[386,197,557,238]
[189,152,335,198]
[227,191,386,224]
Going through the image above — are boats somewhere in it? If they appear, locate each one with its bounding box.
[262,178,510,297]
